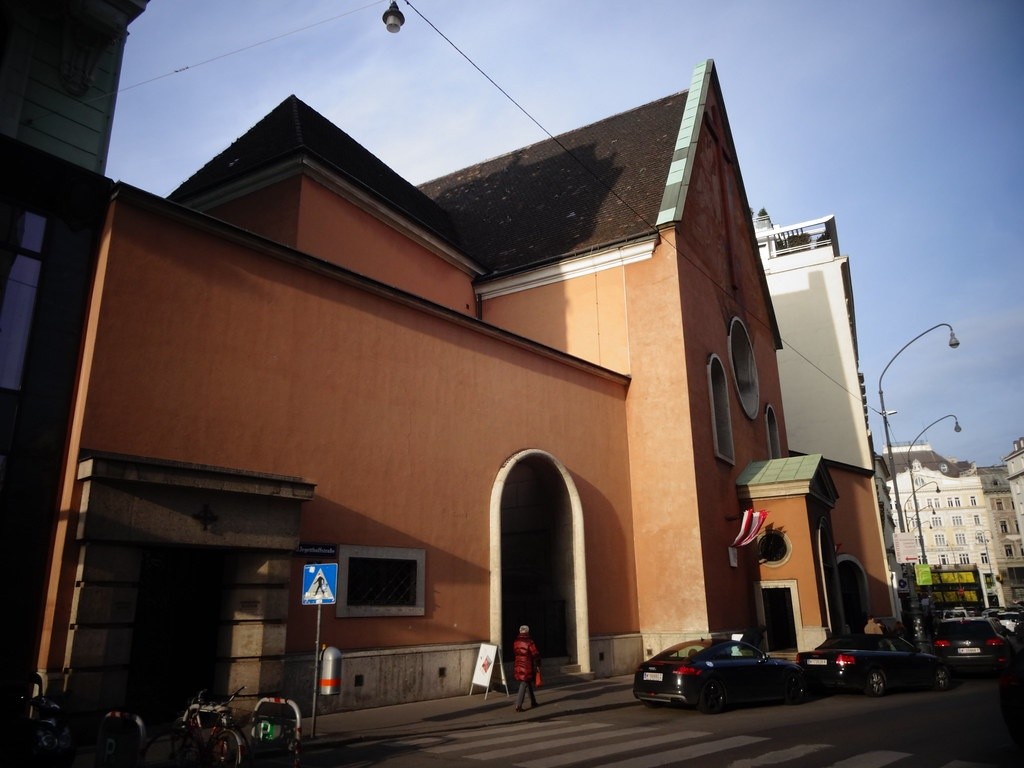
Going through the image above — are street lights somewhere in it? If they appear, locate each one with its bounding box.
[908,415,962,616]
[879,324,960,652]
[904,481,940,535]
[975,530,994,583]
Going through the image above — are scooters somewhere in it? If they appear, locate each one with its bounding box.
[14,669,81,768]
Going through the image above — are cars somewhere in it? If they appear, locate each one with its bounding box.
[633,639,810,715]
[796,634,950,697]
[933,606,1024,632]
[930,616,1015,678]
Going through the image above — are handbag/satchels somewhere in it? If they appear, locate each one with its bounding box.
[535,668,544,687]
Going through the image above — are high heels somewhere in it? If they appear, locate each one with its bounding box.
[531,702,538,707]
[516,705,525,712]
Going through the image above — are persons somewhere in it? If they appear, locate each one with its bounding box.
[945,612,950,618]
[864,615,882,634]
[932,615,940,634]
[893,621,908,641]
[960,612,965,617]
[514,625,542,712]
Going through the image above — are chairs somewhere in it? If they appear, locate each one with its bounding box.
[688,649,697,657]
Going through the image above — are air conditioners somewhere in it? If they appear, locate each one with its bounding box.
[898,578,909,592]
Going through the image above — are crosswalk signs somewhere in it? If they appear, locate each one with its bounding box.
[303,562,338,604]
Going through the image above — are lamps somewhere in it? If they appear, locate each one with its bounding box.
[382,2,405,33]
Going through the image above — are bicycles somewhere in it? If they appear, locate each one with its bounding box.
[142,684,252,768]
